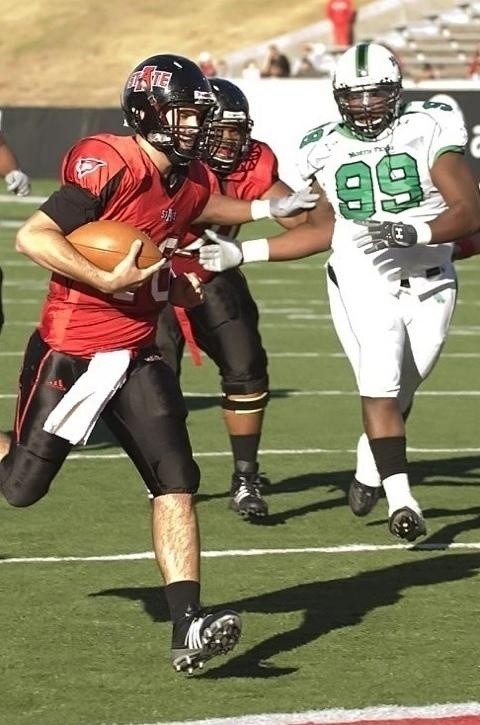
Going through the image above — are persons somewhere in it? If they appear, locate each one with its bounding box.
[1,55,319,672]
[204,41,480,85]
[158,82,310,521]
[199,45,478,543]
[0,107,31,330]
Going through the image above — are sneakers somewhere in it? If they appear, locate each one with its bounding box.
[349,478,382,517]
[228,461,270,517]
[169,610,243,673]
[389,506,429,543]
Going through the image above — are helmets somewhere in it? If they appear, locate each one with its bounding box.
[120,54,216,165]
[203,78,253,172]
[332,43,402,135]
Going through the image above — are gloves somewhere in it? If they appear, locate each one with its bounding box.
[353,216,432,253]
[3,171,32,197]
[199,227,271,273]
[250,187,320,222]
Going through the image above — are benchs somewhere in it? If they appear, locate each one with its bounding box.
[260,2,479,82]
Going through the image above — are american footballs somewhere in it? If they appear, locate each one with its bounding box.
[66,220,164,272]
